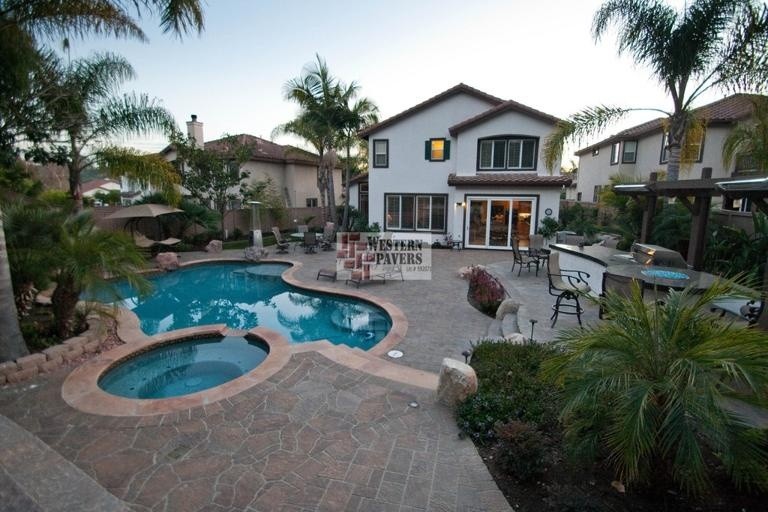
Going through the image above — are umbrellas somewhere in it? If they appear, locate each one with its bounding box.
[102,201,187,236]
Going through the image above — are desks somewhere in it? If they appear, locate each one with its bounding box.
[606,265,733,320]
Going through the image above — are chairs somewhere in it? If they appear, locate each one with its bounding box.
[710,293,767,392]
[126,227,183,258]
[599,273,664,330]
[272,221,335,255]
[547,252,591,332]
[505,232,623,276]
